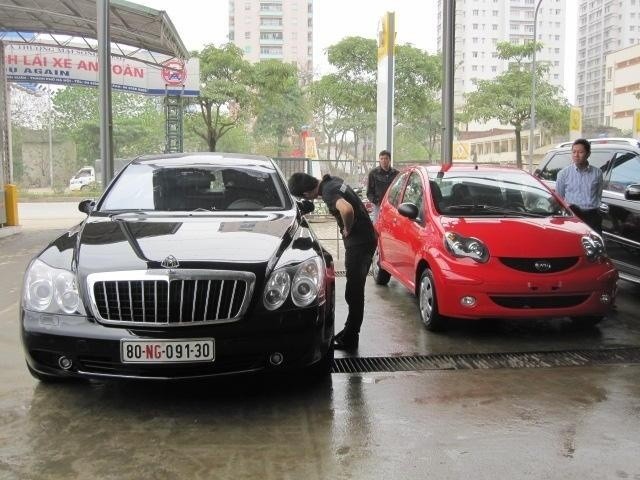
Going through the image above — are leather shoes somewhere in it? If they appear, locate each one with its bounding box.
[333,340,359,349]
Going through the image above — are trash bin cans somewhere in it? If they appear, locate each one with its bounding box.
[0,189,7,228]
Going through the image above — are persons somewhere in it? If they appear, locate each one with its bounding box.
[288,172,378,348]
[556,139,602,236]
[366,150,400,225]
